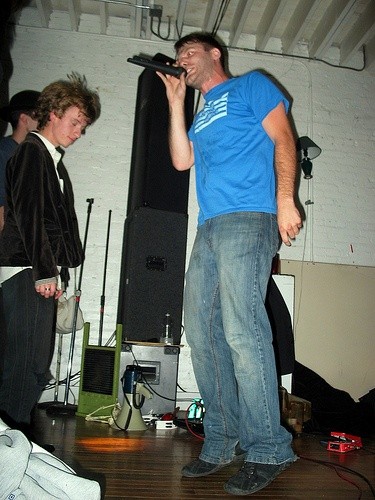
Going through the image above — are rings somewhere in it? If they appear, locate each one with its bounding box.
[45,288,50,290]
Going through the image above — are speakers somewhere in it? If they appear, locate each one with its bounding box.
[127,67,195,213]
[115,207,188,345]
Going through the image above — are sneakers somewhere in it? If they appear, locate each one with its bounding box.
[223,448,294,495]
[180,441,250,477]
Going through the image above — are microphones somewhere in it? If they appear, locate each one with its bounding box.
[126,58,188,78]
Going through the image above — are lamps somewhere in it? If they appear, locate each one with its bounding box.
[296,136,322,179]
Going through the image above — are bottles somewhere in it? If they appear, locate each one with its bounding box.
[159,311,173,346]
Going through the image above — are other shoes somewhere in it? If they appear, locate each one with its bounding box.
[12,423,55,453]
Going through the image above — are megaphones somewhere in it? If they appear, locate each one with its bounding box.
[109,365,152,431]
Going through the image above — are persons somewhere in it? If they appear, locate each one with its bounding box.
[157,32,303,495]
[0,89,44,229]
[0,80,102,451]
